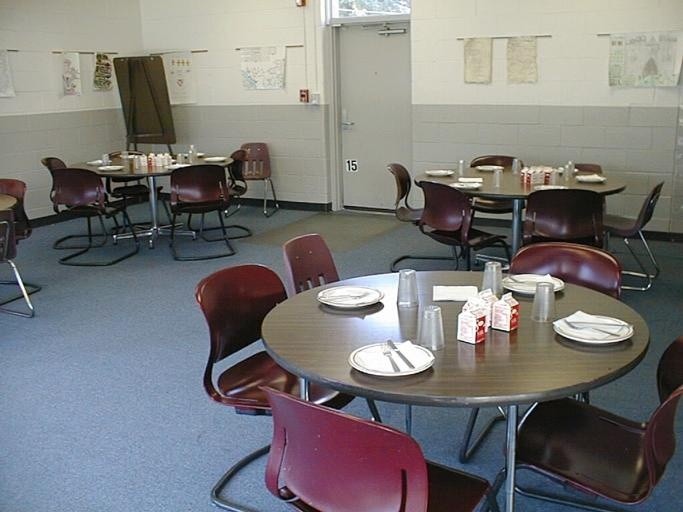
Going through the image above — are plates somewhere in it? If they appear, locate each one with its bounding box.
[552,312,636,347]
[204,156,226,162]
[575,173,609,184]
[96,165,127,173]
[347,343,436,377]
[505,274,564,298]
[319,285,384,309]
[448,182,483,189]
[426,169,454,176]
[476,165,504,172]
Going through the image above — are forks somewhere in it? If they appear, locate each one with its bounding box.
[563,319,622,338]
[509,276,538,286]
[319,293,367,299]
[383,348,401,373]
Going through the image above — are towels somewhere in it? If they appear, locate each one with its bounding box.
[505,273,561,292]
[329,286,381,304]
[552,309,624,342]
[432,286,478,306]
[356,338,435,375]
[459,177,484,182]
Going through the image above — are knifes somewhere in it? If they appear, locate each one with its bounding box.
[386,338,416,368]
[569,318,629,329]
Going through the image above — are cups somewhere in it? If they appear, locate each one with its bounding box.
[459,157,468,178]
[397,268,419,312]
[512,157,521,174]
[481,260,503,297]
[417,305,445,351]
[493,168,505,188]
[533,282,556,324]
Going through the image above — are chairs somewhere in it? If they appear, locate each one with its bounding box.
[105,148,173,233]
[255,377,500,512]
[480,335,682,510]
[224,141,278,220]
[168,162,233,259]
[40,157,106,250]
[283,234,339,292]
[416,179,510,269]
[0,177,41,303]
[193,147,252,237]
[602,180,665,292]
[193,270,379,507]
[574,162,604,175]
[524,188,602,237]
[456,243,619,462]
[56,167,139,267]
[470,153,528,248]
[0,208,35,320]
[388,164,459,272]
